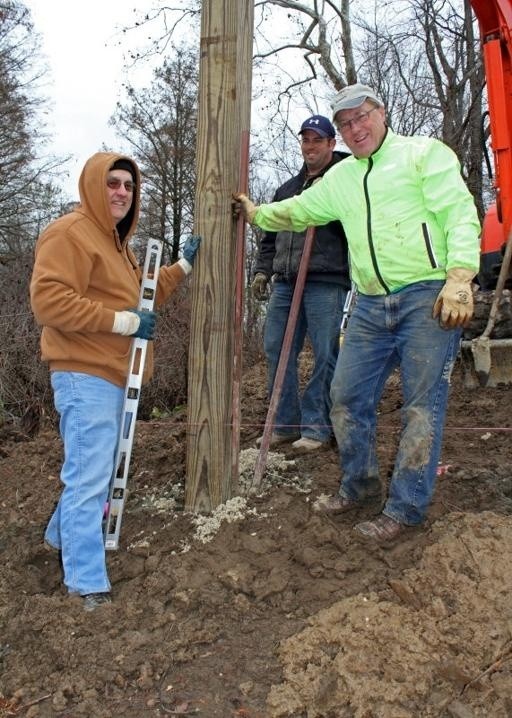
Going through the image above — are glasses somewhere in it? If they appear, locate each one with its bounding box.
[336,108,375,132]
[107,177,135,192]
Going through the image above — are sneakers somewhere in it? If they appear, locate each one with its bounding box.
[355,516,401,545]
[254,432,297,445]
[311,494,358,516]
[72,593,114,610]
[292,436,324,452]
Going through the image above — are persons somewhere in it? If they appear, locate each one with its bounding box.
[252,115,356,454]
[30,153,202,611]
[233,84,482,546]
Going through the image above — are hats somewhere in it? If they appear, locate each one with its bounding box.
[297,115,335,138]
[331,83,384,123]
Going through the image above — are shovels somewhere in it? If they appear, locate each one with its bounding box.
[470,238,512,387]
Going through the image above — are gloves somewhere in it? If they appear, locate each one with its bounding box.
[230,192,257,220]
[116,307,157,340]
[183,235,200,264]
[432,268,479,328]
[250,273,269,301]
